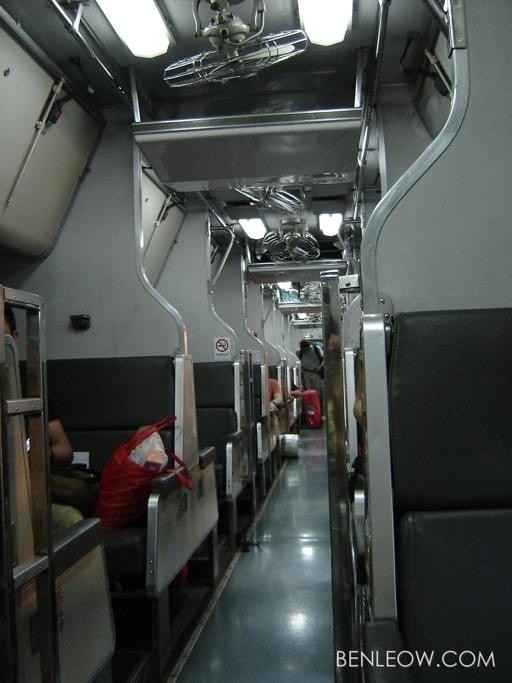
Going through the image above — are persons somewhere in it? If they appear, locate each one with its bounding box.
[267,377,284,433]
[299,339,327,420]
[288,381,301,397]
[351,349,367,469]
[1,299,85,537]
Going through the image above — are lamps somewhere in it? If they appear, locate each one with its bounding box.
[95,1,175,61]
[298,0,355,47]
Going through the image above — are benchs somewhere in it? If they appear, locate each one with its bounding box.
[0,309,512,683]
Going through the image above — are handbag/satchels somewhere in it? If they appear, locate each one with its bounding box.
[96,415,195,531]
[46,465,100,514]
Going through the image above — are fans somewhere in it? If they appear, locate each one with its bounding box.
[163,0,326,324]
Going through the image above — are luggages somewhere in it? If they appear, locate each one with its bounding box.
[302,389,321,429]
